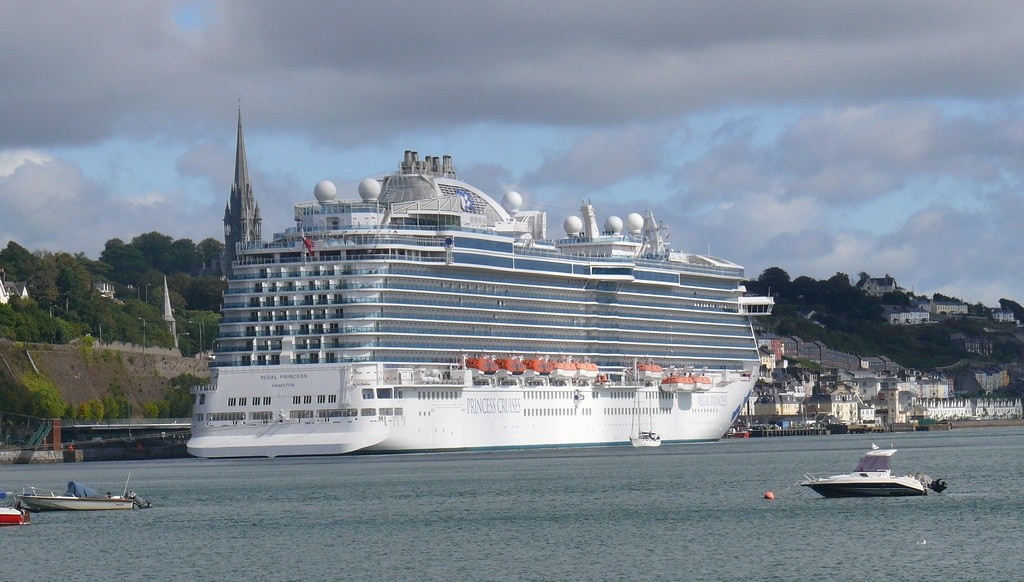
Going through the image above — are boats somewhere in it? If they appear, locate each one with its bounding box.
[661,374,696,393]
[630,361,665,380]
[459,357,500,381]
[16,482,153,511]
[495,358,528,379]
[572,360,600,380]
[548,360,578,381]
[0,504,32,525]
[689,375,714,392]
[520,360,553,378]
[801,447,949,497]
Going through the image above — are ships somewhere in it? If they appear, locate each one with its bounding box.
[184,149,778,459]
